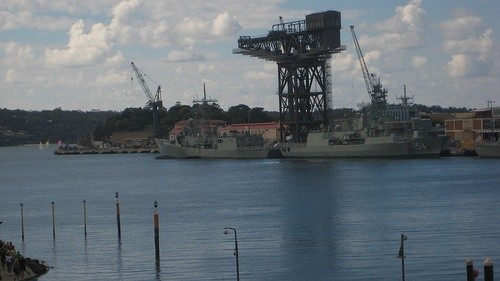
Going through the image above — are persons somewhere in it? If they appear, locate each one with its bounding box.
[0,239,26,280]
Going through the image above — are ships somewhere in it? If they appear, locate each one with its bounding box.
[158,82,282,158]
[476,138,500,157]
[280,76,453,157]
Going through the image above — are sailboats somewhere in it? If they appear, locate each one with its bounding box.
[39,140,62,151]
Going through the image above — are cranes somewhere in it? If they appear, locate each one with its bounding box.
[129,61,163,125]
[349,25,387,111]
